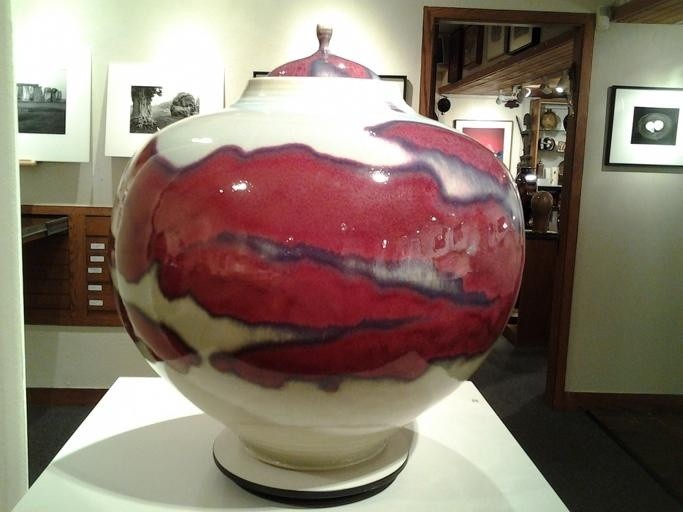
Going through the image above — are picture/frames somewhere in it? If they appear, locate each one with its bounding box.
[448,24,540,82]
[454,121,514,174]
[603,86,682,167]
[253,71,407,109]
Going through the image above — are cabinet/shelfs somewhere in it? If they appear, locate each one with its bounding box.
[530,98,568,187]
